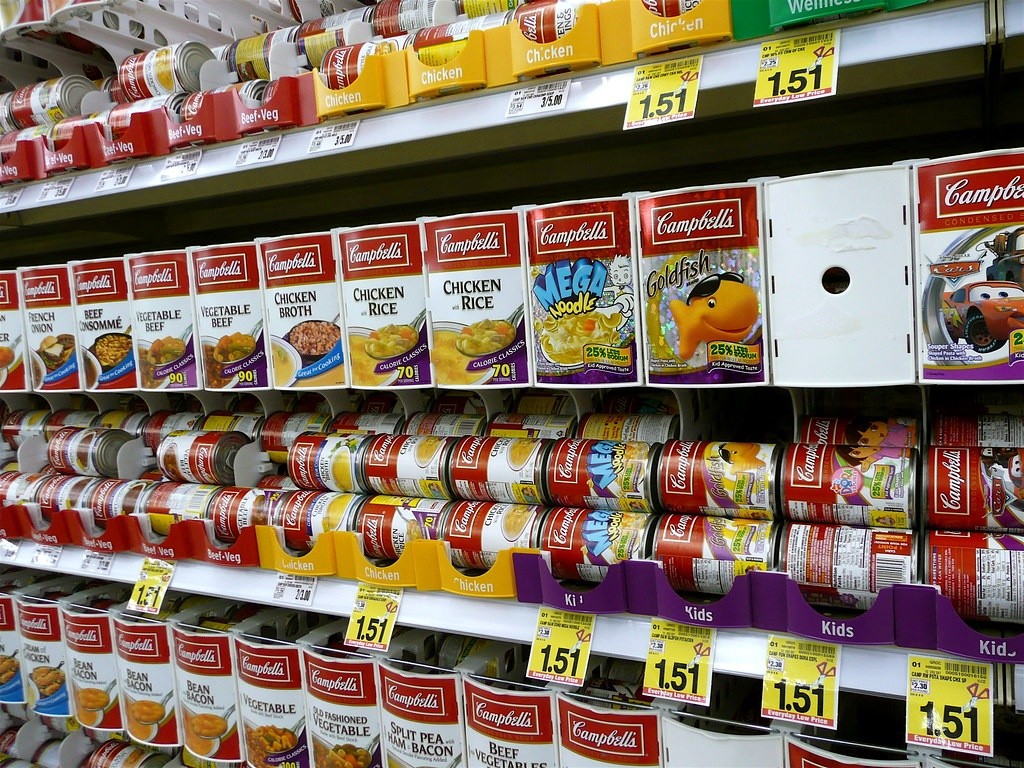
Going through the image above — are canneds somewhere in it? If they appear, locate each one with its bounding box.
[0,726,175,768]
[0,0,701,156]
[0,396,1024,630]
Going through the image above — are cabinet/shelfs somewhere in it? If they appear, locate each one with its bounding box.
[1,0,1023,768]
